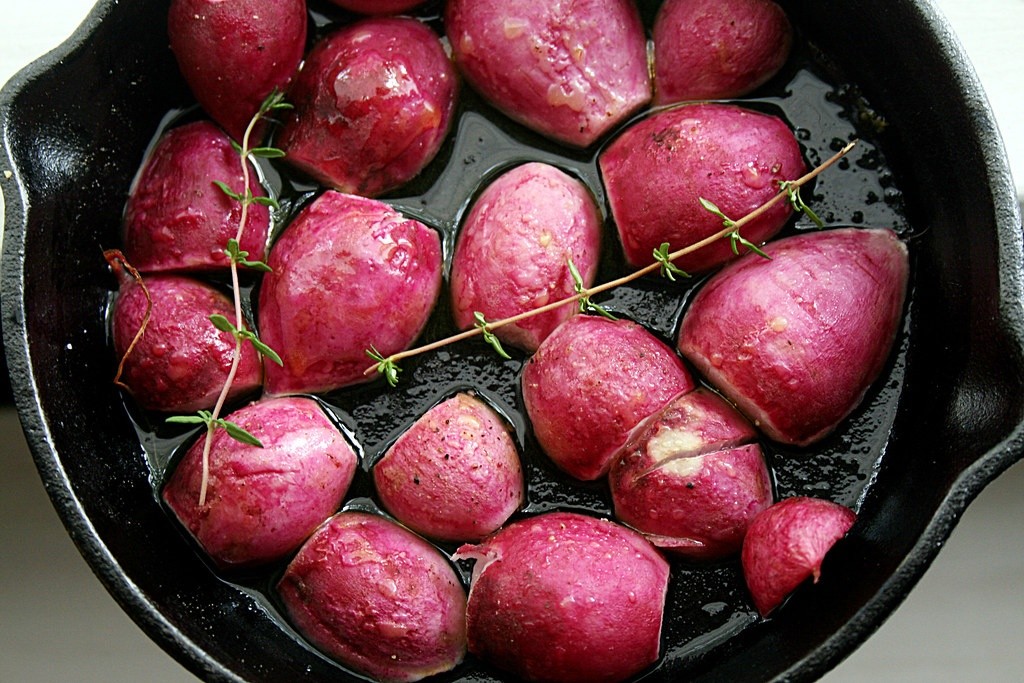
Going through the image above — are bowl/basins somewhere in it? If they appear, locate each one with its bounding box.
[2,0,1024,683]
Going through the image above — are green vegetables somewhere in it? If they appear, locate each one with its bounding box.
[363,142,855,388]
[166,89,297,504]
